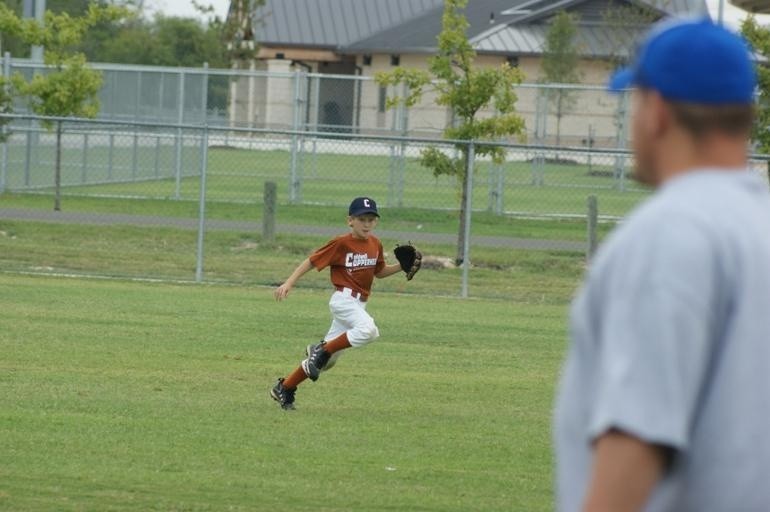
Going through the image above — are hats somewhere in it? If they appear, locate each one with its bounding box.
[349,197,380,218]
[609,14,758,104]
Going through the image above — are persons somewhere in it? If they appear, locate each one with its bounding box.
[553,14,768,511]
[268,197,423,412]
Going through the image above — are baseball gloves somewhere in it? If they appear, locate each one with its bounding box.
[394,246,421,280]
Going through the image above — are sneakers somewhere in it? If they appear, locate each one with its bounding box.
[270,377,297,410]
[301,340,331,382]
